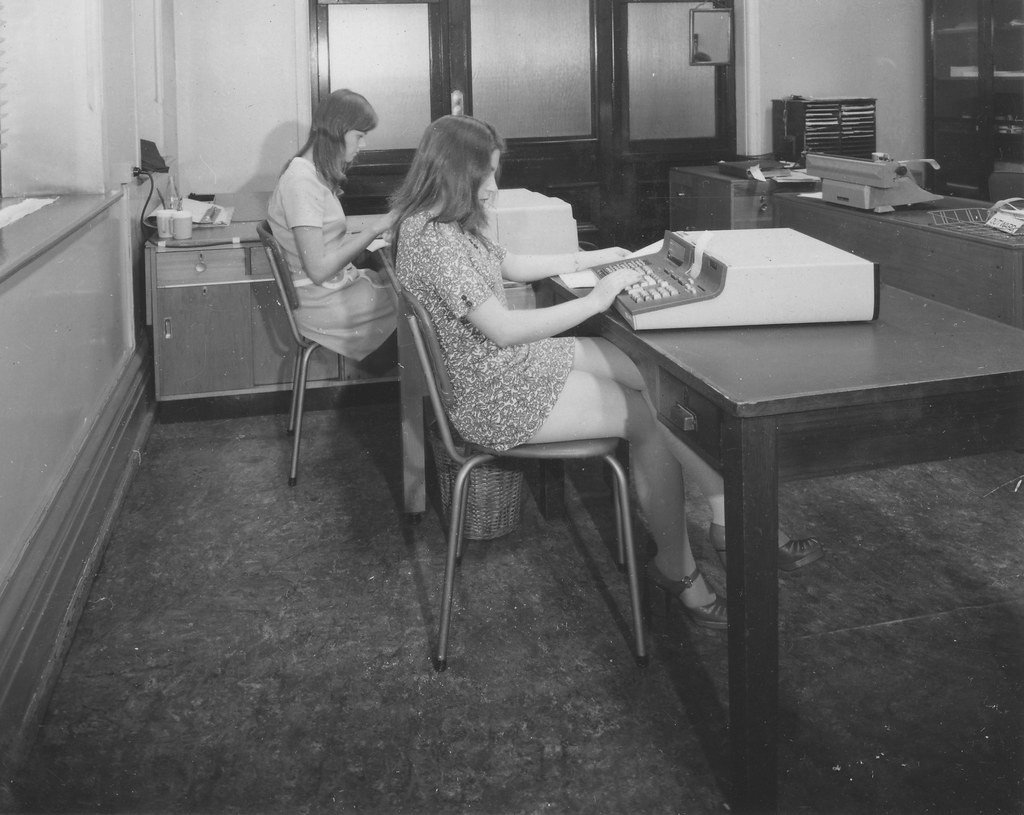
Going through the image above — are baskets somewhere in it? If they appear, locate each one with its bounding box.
[427,414,528,542]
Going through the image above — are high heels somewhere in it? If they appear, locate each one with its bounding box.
[709,522,826,572]
[644,554,728,630]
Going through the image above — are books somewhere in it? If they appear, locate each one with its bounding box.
[717,159,821,183]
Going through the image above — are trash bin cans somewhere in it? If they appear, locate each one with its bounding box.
[429,420,524,541]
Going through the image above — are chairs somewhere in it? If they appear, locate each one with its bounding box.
[257,220,396,487]
[377,251,647,674]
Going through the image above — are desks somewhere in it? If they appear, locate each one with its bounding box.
[536,260,1024,815]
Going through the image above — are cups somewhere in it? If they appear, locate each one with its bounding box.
[156,209,192,240]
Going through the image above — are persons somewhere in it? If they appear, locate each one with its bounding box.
[394,115,825,628]
[266,90,399,363]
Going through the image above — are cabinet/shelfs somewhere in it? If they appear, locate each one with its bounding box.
[145,190,400,400]
[669,166,923,233]
[925,0,1024,205]
[770,97,878,167]
[772,191,1024,329]
